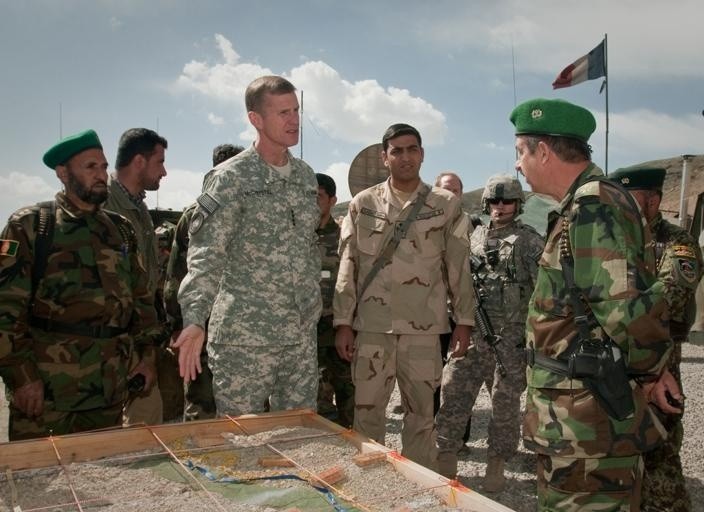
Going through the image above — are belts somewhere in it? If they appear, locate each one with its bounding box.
[521,346,572,376]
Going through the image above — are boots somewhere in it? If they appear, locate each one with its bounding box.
[436,450,458,480]
[482,456,507,494]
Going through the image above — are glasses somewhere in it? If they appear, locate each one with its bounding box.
[486,197,519,205]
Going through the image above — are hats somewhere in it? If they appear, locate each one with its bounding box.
[42,129,104,170]
[609,165,666,191]
[509,98,597,143]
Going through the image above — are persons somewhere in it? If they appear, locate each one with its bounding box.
[333,124,475,471]
[311,174,354,431]
[510,99,684,511]
[172,76,320,420]
[435,174,480,455]
[102,129,166,432]
[609,167,701,511]
[432,177,544,495]
[163,144,246,422]
[0,129,157,441]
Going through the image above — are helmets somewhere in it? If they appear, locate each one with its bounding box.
[480,174,526,214]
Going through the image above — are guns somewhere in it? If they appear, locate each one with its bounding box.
[473,288,506,380]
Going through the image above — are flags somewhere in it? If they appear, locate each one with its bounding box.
[552,38,607,90]
